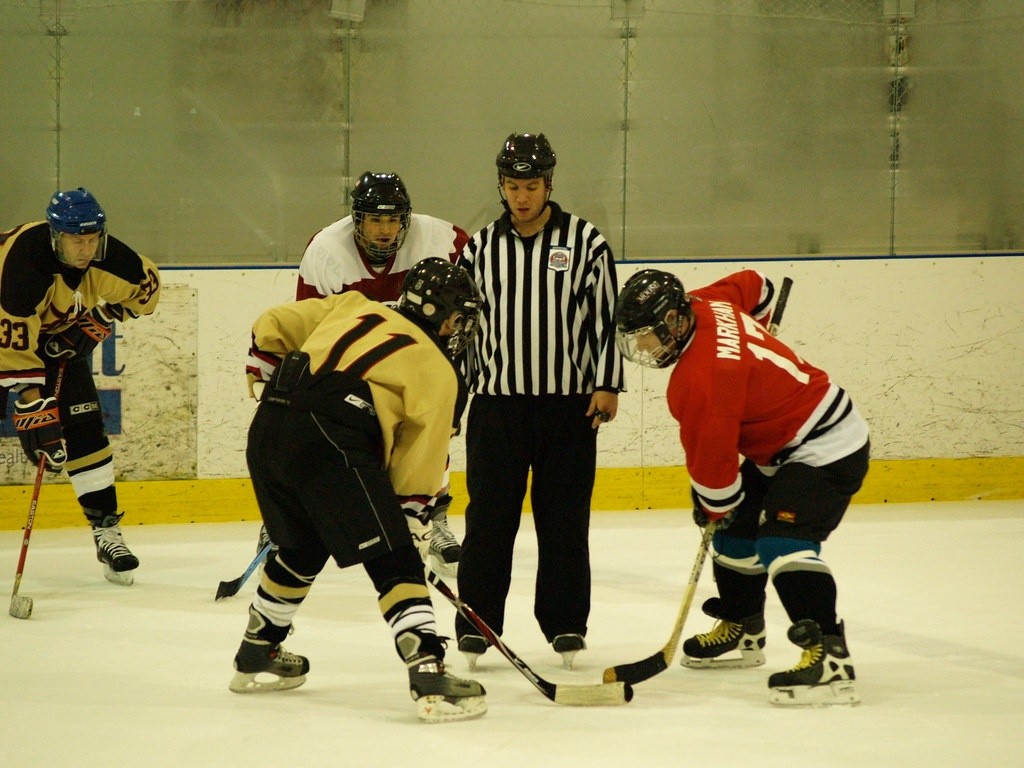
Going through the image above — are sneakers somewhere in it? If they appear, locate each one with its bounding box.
[230,642,310,692]
[680,598,765,668]
[395,629,486,722]
[768,620,862,706]
[423,497,460,569]
[458,633,489,670]
[90,512,139,585]
[552,633,587,671]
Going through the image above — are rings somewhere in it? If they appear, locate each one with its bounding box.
[596,410,610,422]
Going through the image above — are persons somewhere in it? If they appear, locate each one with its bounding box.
[0,188,160,585]
[230,257,487,722]
[256,171,470,576]
[612,269,872,707]
[454,131,629,669]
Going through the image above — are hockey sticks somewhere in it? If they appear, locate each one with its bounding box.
[254,377,635,707]
[214,525,276,601]
[603,274,795,687]
[8,366,67,620]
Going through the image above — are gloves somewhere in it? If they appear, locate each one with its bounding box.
[13,397,68,473]
[694,507,736,530]
[54,305,114,353]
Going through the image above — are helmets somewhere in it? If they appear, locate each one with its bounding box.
[616,269,690,368]
[351,170,412,264]
[496,132,556,178]
[404,257,483,356]
[46,188,106,235]
